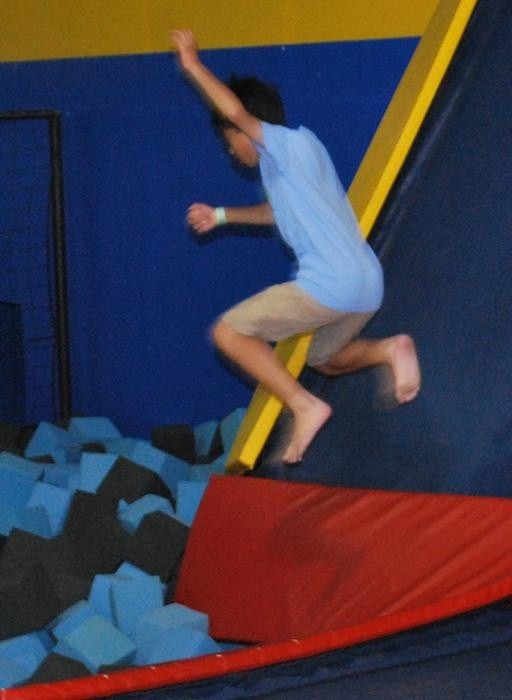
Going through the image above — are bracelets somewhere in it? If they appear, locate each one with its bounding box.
[214,204,227,228]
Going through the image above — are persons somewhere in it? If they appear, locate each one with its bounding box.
[170,26,421,464]
